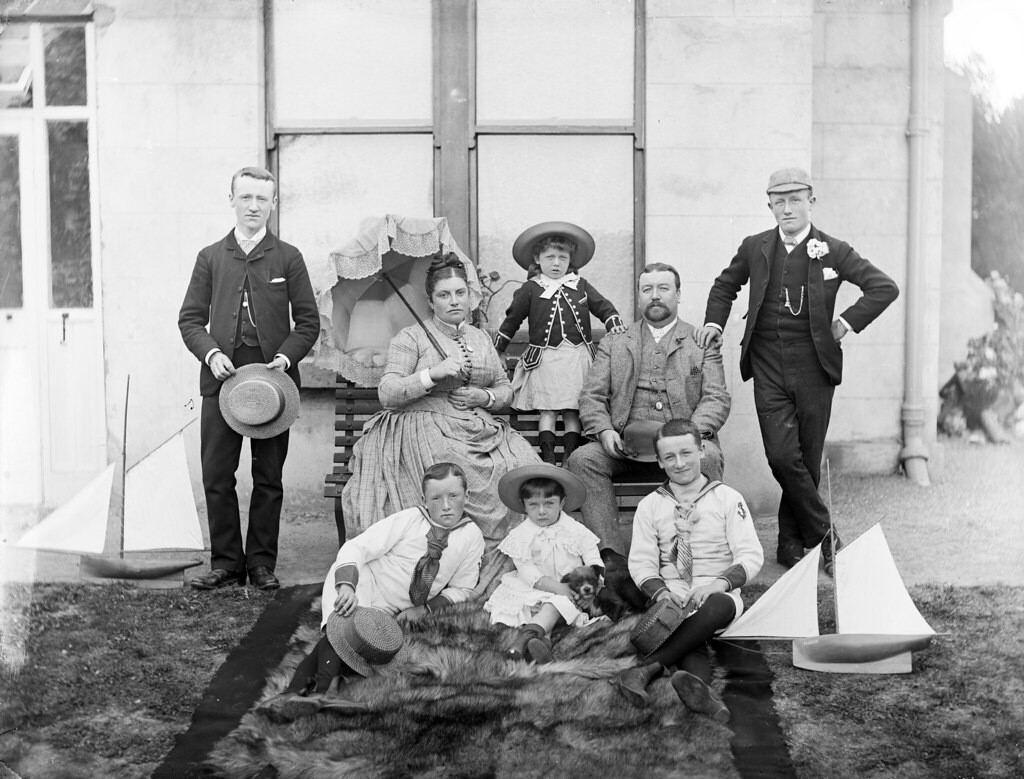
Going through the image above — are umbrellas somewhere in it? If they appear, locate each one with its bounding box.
[313,214,484,388]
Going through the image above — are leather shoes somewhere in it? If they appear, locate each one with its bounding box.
[189,568,239,589]
[248,566,280,591]
[671,670,730,723]
[607,659,662,708]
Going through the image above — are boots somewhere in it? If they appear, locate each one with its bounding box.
[539,430,557,466]
[561,432,581,469]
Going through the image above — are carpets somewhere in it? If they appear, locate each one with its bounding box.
[1,582,1024,779]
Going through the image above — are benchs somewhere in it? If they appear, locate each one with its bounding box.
[325,359,668,548]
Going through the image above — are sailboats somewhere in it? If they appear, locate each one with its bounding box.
[710,459,952,674]
[15,375,210,579]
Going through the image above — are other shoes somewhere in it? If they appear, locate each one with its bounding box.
[255,691,369,725]
[505,624,555,664]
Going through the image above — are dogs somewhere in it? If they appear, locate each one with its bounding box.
[561,548,654,622]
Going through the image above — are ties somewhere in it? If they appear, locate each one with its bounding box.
[668,505,700,584]
[409,526,449,607]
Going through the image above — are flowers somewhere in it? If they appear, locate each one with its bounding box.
[806,238,830,261]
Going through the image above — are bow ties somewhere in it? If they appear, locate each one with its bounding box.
[782,237,799,246]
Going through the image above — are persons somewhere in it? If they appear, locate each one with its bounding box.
[692,168,899,578]
[483,464,605,663]
[341,252,544,600]
[179,168,321,590]
[607,418,764,723]
[256,462,485,720]
[566,262,732,571]
[494,222,629,466]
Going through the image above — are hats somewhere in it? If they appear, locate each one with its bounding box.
[219,363,300,439]
[512,221,595,269]
[498,465,588,514]
[630,597,693,661]
[326,606,409,679]
[615,420,665,462]
[766,168,812,194]
